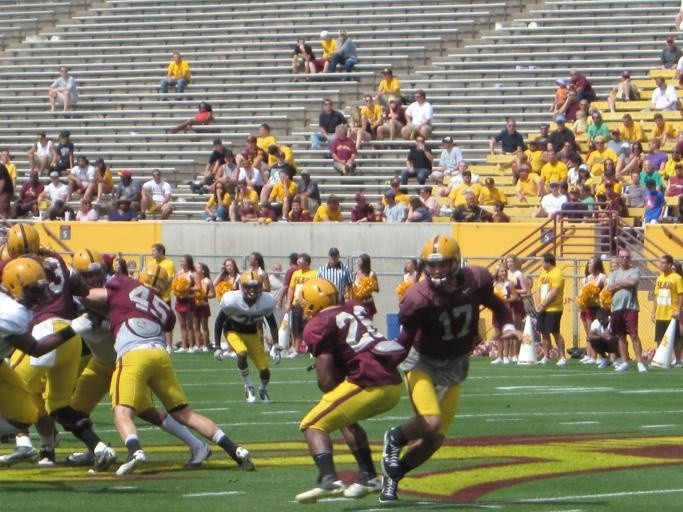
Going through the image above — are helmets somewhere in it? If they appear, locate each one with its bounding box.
[0,244,10,263]
[237,270,262,301]
[2,257,47,309]
[7,223,39,259]
[420,234,461,286]
[71,248,106,281]
[298,277,339,321]
[137,265,168,296]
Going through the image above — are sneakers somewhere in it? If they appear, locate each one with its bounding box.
[88,447,117,472]
[615,362,629,371]
[294,474,343,500]
[381,426,403,481]
[192,345,200,351]
[229,351,237,358]
[244,386,255,403]
[232,445,254,471]
[676,360,682,366]
[288,350,297,358]
[174,347,187,353]
[670,358,676,367]
[512,355,517,361]
[582,358,596,365]
[188,347,193,352]
[555,358,566,365]
[115,449,145,475]
[223,351,228,356]
[36,445,55,465]
[580,355,590,362]
[377,474,400,502]
[502,356,509,364]
[0,447,38,467]
[166,345,171,354]
[343,473,383,497]
[611,360,618,368]
[638,362,646,373]
[184,442,211,465]
[539,356,550,364]
[68,449,92,464]
[54,430,59,448]
[598,359,609,368]
[490,357,502,364]
[258,389,269,401]
[202,344,207,352]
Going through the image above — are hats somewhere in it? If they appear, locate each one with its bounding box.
[388,96,395,102]
[329,247,338,255]
[337,29,345,37]
[117,170,131,178]
[390,178,398,185]
[494,201,502,206]
[245,135,256,143]
[31,171,37,177]
[596,191,604,196]
[320,30,326,38]
[485,179,494,184]
[605,180,609,184]
[94,159,104,164]
[644,178,654,182]
[50,171,57,177]
[442,136,452,144]
[621,143,629,147]
[569,187,578,192]
[578,165,586,170]
[556,115,564,122]
[287,253,296,258]
[620,72,629,77]
[115,195,130,204]
[354,193,366,201]
[380,69,390,73]
[301,169,309,175]
[549,180,556,185]
[384,192,392,197]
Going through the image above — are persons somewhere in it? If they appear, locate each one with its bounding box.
[0,243,39,465]
[5,224,115,471]
[0,149,17,190]
[407,195,433,223]
[675,1,683,33]
[36,171,69,221]
[112,257,129,275]
[48,199,75,221]
[375,67,401,104]
[660,36,683,71]
[504,254,528,362]
[160,51,192,100]
[295,278,403,501]
[608,70,640,112]
[139,169,173,220]
[671,262,683,366]
[48,66,78,111]
[361,95,383,129]
[268,252,317,358]
[213,271,281,404]
[13,171,44,219]
[75,199,98,221]
[65,248,211,468]
[165,102,212,133]
[401,258,423,285]
[650,255,683,368]
[313,195,345,223]
[349,104,374,150]
[490,263,517,365]
[319,247,353,302]
[523,275,538,320]
[147,244,176,353]
[535,253,567,366]
[1,258,94,439]
[173,254,195,354]
[419,185,440,216]
[376,96,406,150]
[381,191,406,225]
[430,136,463,183]
[379,235,523,504]
[73,264,257,477]
[578,256,619,369]
[640,74,678,113]
[82,157,114,201]
[549,71,595,119]
[330,29,358,80]
[309,99,348,150]
[291,38,315,81]
[589,310,620,369]
[49,131,74,174]
[192,261,216,353]
[438,160,510,223]
[400,135,433,185]
[114,169,141,201]
[0,161,13,220]
[187,123,321,223]
[607,249,647,372]
[350,192,375,223]
[248,251,272,348]
[329,125,358,175]
[109,198,139,223]
[512,109,683,224]
[382,178,409,204]
[489,118,523,155]
[28,131,57,175]
[310,30,337,73]
[400,89,432,139]
[676,56,683,86]
[69,155,94,197]
[351,253,379,322]
